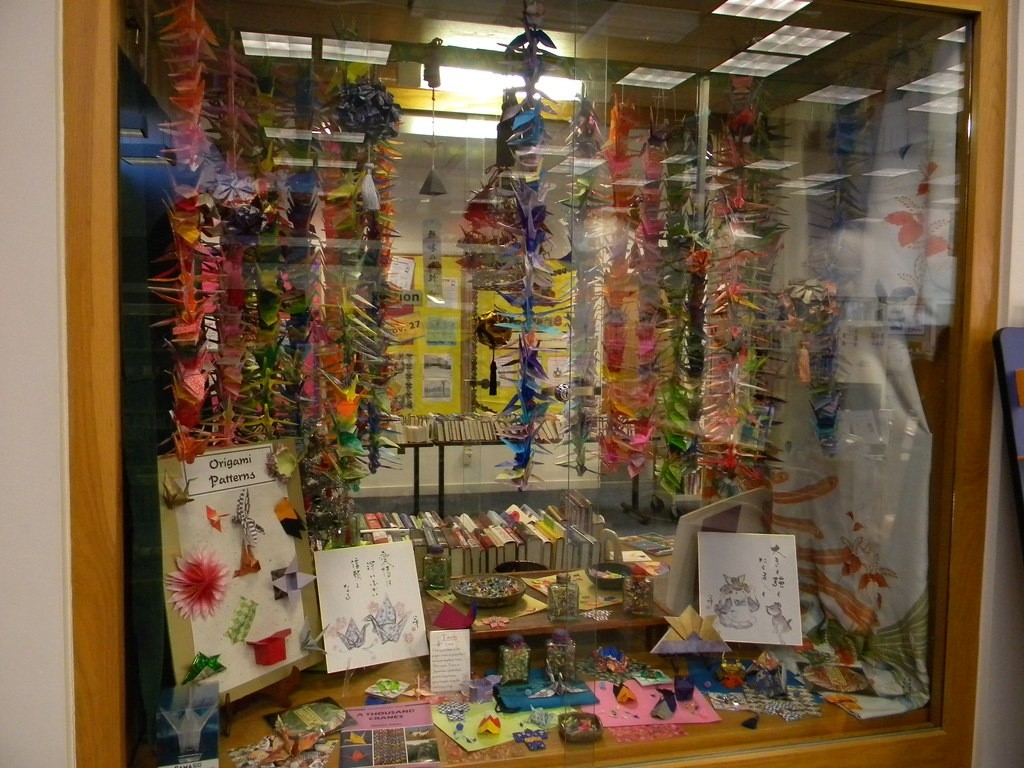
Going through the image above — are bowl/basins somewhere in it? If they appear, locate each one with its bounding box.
[585,562,632,590]
[452,573,526,608]
[558,712,604,743]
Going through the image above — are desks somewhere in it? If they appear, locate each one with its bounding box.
[363,436,661,525]
[420,561,671,652]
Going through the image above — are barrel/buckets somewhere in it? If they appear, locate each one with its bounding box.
[493,560,548,574]
